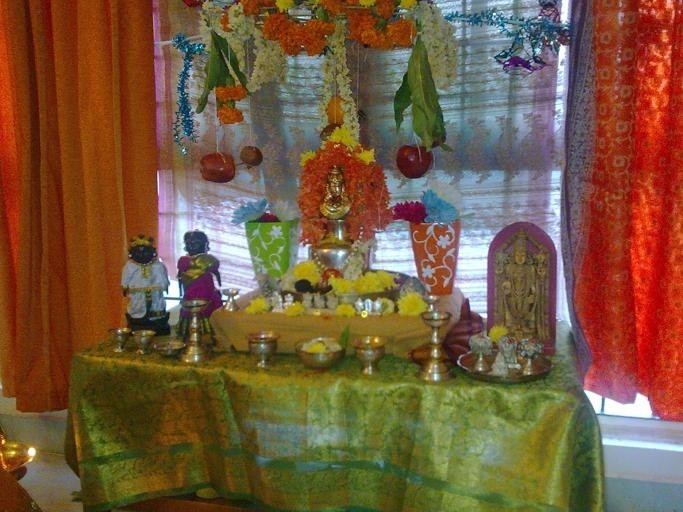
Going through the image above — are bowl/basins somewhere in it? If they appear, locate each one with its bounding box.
[294,340,345,368]
[152,341,186,356]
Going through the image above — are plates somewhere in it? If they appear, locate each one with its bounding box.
[457,348,553,384]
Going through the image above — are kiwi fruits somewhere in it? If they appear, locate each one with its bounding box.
[240,145,263,169]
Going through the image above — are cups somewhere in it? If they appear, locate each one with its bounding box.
[352,334,386,376]
[132,330,156,354]
[246,331,280,369]
[110,328,131,352]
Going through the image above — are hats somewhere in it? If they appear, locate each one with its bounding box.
[127,233,154,249]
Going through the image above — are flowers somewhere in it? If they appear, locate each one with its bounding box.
[229,198,302,226]
[326,270,400,295]
[281,300,305,317]
[334,303,356,318]
[389,188,458,228]
[377,297,394,318]
[303,342,327,354]
[487,324,508,342]
[280,259,342,295]
[395,291,427,316]
[244,295,271,315]
[181,1,460,153]
[294,124,394,250]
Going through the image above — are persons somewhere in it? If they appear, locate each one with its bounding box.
[501,239,537,336]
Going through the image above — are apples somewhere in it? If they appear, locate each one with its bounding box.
[396,144,432,178]
[199,152,243,183]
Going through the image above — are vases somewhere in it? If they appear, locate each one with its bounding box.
[243,222,293,293]
[312,219,353,270]
[408,219,461,296]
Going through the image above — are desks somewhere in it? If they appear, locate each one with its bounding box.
[72,320,583,510]
